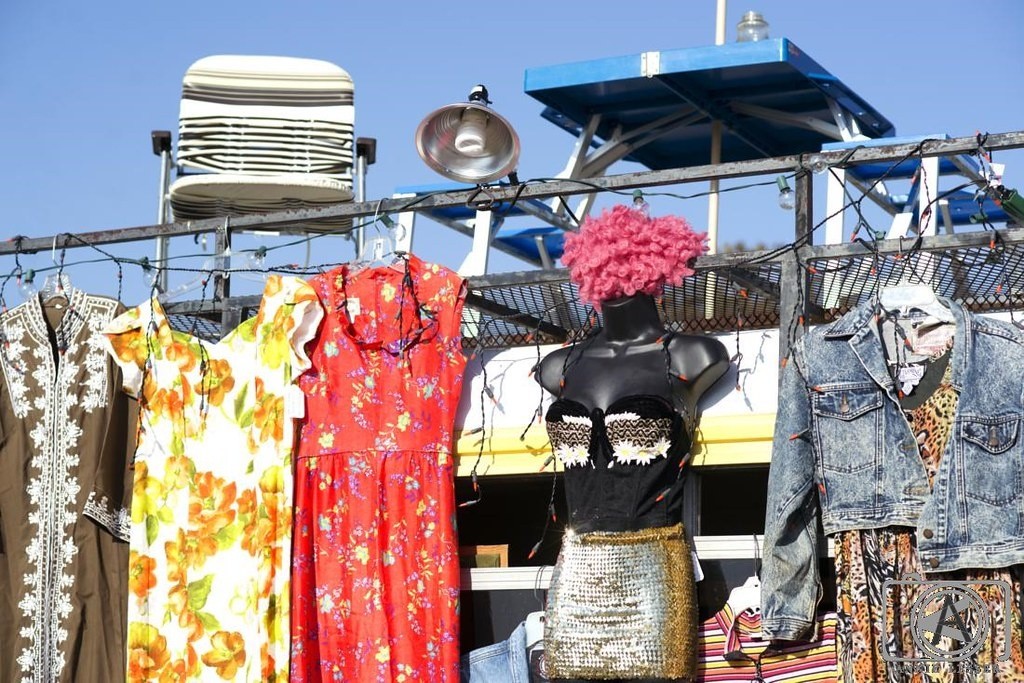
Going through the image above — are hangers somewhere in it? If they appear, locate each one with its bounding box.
[0,195,1023,357]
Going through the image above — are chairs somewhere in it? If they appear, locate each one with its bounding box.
[149,54,376,303]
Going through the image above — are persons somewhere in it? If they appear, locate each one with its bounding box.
[530,291,733,681]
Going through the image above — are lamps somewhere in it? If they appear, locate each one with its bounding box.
[414,82,523,189]
